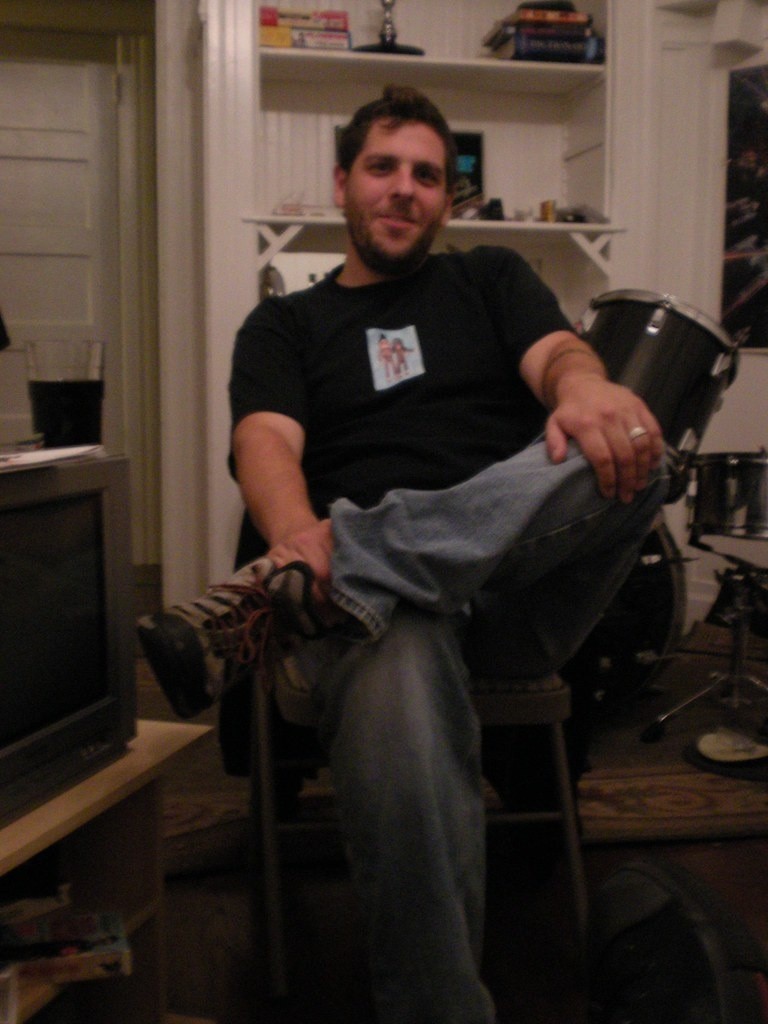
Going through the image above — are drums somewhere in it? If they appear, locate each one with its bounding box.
[690,448,768,572]
[578,289,739,505]
[561,507,688,717]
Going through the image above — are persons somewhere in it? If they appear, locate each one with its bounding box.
[133,84,671,1023]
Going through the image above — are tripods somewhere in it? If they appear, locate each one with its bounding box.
[643,571,768,743]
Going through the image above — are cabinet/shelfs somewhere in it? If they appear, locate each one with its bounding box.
[0,719,214,1022]
[252,0,614,329]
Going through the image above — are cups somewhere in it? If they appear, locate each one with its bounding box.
[28,339,107,450]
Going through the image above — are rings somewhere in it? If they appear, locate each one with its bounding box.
[628,427,648,441]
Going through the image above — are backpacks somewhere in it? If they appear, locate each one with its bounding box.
[595,858,768,1024]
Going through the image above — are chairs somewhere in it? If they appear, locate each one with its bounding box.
[262,688,590,1023]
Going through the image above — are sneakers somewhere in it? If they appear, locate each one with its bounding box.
[137,556,344,718]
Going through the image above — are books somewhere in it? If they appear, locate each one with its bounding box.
[483,9,605,64]
[259,6,352,50]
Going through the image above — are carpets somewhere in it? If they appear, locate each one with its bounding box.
[159,759,768,879]
